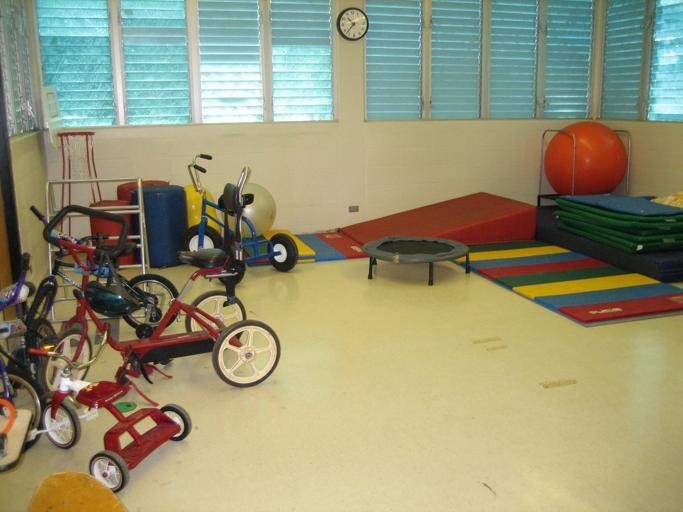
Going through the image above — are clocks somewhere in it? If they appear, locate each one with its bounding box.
[337,8,369,41]
